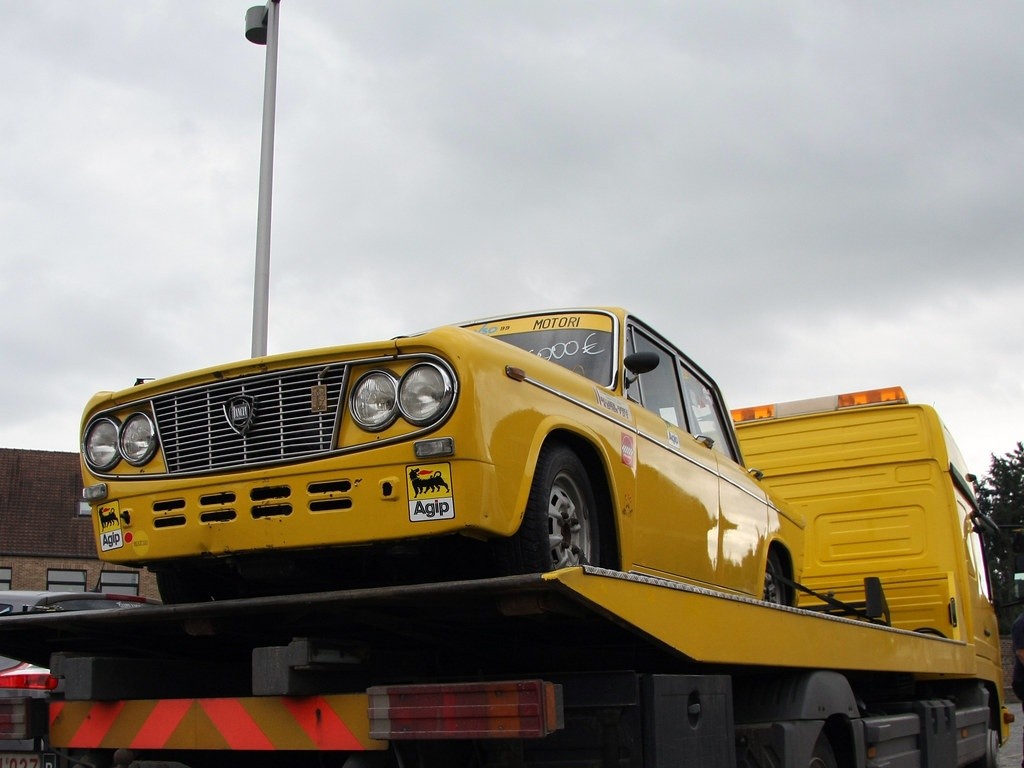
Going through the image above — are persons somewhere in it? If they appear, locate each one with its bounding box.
[1012,609,1024,768]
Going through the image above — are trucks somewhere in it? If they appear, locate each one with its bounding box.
[0,385,1024,767]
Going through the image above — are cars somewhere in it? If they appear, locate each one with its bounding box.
[0,590,162,768]
[77,304,808,608]
